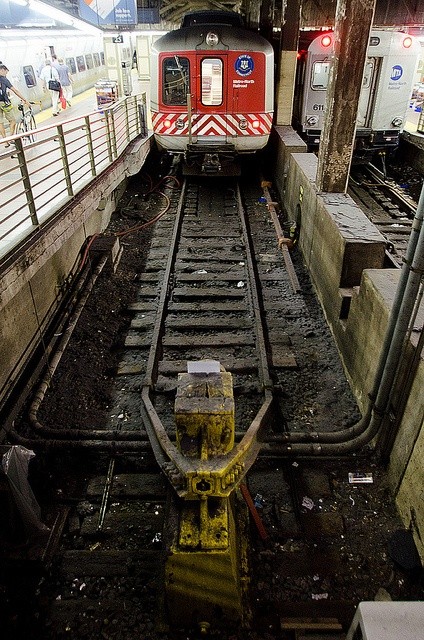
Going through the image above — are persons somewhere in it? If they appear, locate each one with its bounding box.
[55,59,74,108]
[51,54,60,71]
[0,65,31,148]
[38,60,61,116]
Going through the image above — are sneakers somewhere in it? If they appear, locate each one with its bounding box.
[5,143,10,148]
[53,114,58,117]
[57,112,59,114]
[10,140,15,144]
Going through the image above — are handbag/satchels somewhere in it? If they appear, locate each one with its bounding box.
[48,79,61,91]
[0,90,14,112]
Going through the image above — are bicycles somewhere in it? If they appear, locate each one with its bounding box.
[15,100,37,149]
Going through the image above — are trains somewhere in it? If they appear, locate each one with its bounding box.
[293,30,420,165]
[0,29,136,128]
[150,11,275,178]
